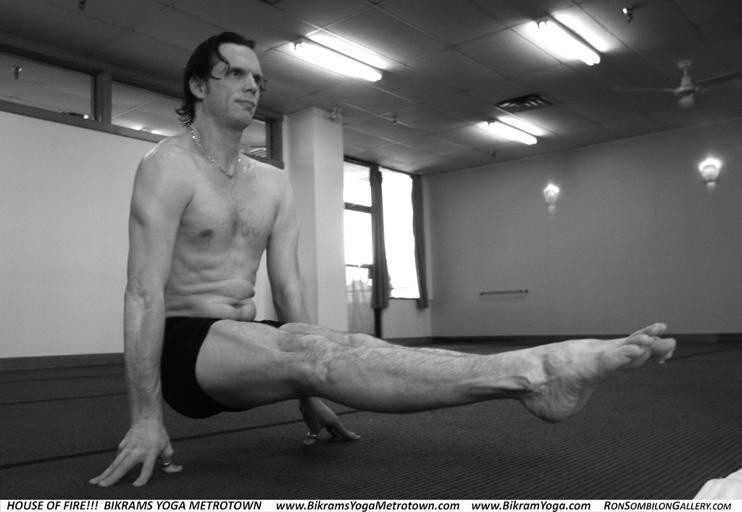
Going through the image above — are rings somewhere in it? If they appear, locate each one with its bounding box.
[306,432,320,439]
[159,462,173,466]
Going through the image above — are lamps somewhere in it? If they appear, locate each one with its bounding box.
[291,38,384,85]
[535,16,601,68]
[698,153,721,188]
[488,118,540,147]
[544,180,561,212]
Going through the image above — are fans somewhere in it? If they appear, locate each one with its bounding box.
[598,58,740,110]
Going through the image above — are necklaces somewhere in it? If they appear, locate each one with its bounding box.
[190,124,240,180]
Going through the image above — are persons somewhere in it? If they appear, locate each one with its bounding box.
[88,32,676,489]
